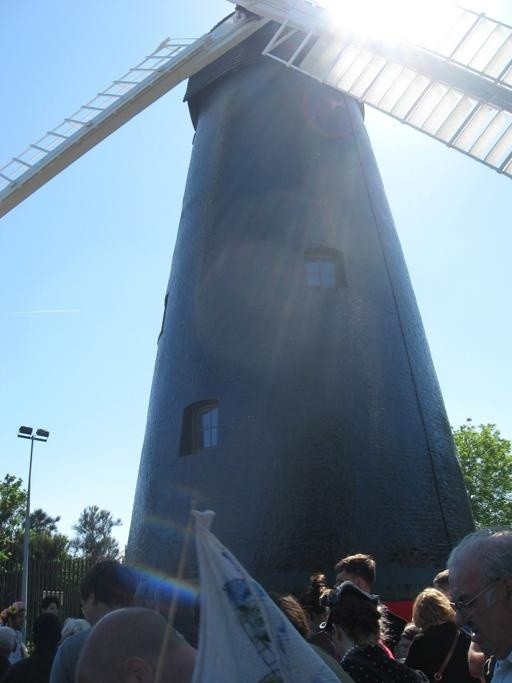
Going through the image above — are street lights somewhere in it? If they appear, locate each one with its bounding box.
[17,424,50,645]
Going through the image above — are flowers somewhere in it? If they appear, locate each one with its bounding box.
[0,601,25,625]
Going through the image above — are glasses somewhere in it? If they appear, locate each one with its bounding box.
[450,581,496,616]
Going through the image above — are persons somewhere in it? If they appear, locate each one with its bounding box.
[270,555,487,681]
[448,530,512,683]
[0,561,202,683]
